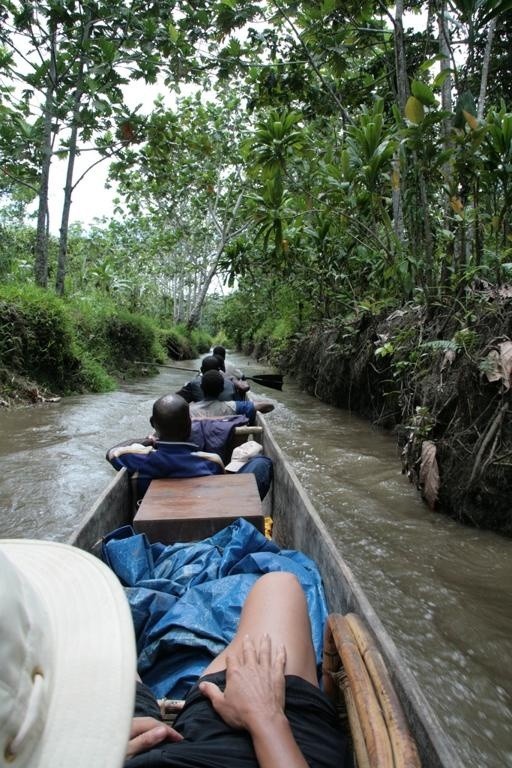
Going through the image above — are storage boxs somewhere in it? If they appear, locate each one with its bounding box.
[132,472,265,544]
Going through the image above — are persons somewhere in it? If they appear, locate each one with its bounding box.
[106,394,275,502]
[189,369,275,418]
[0,537,353,768]
[213,355,250,391]
[176,356,242,403]
[213,346,244,379]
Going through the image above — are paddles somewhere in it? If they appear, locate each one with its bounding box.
[134,361,284,392]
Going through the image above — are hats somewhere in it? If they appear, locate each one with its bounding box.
[1,533,136,768]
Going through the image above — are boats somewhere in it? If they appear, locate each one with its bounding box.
[34,347,471,768]
